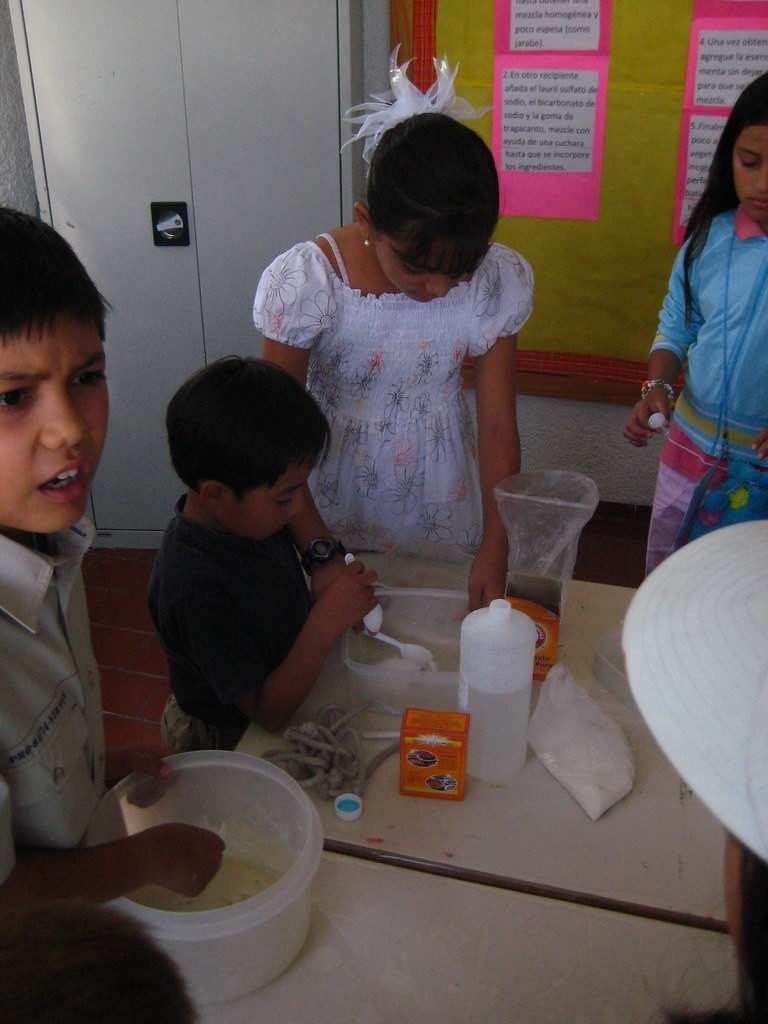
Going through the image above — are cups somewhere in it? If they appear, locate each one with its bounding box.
[492,469,599,620]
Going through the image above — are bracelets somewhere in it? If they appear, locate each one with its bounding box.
[641,379,674,400]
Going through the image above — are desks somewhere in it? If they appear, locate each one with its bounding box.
[196,555,738,1024]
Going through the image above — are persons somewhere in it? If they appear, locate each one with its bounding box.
[253,98,534,621]
[0,206,225,1024]
[622,71,768,577]
[147,354,380,761]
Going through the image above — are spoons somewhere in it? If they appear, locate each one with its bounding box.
[362,626,433,664]
[344,552,383,633]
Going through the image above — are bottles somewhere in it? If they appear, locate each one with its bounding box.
[455,599,536,784]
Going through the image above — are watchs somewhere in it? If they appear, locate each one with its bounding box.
[300,537,347,577]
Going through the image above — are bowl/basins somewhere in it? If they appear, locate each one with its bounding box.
[83,749,325,1004]
[341,587,483,682]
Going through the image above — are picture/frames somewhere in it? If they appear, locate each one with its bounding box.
[387,0,768,410]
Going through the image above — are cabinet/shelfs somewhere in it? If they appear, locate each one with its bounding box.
[8,0,364,547]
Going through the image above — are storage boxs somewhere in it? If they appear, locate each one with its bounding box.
[399,708,470,801]
[503,571,564,680]
[340,586,470,713]
